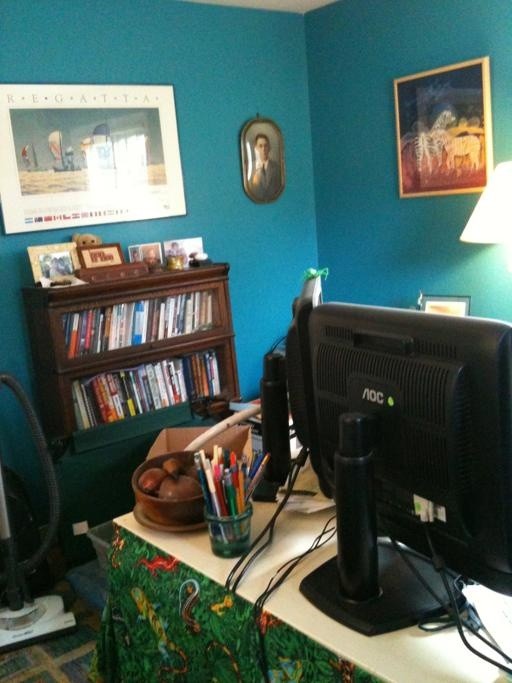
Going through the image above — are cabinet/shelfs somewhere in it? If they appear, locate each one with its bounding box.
[20,263,242,452]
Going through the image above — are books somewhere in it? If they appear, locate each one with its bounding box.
[58,285,225,432]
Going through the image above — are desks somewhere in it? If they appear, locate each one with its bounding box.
[90,484,512,683]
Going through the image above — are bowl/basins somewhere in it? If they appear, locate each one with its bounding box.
[131,451,216,526]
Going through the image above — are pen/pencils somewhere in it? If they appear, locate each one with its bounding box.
[193,445,270,557]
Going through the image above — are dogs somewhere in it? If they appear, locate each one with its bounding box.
[433,129,481,170]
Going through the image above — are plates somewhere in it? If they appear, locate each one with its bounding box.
[134,502,211,533]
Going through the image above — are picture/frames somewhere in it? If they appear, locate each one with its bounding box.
[77,243,126,269]
[393,56,495,198]
[239,119,284,204]
[420,296,470,316]
[27,242,81,284]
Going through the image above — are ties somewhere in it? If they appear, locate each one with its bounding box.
[260,163,266,187]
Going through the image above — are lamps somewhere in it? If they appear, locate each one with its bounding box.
[459,161,511,245]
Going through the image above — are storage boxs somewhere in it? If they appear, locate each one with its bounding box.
[146,425,254,474]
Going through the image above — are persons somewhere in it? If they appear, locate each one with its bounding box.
[129,241,187,271]
[246,132,282,200]
[49,256,59,278]
[43,254,51,279]
[58,258,72,276]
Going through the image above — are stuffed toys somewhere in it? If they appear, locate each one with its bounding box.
[71,233,102,248]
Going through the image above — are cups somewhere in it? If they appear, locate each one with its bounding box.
[165,255,184,271]
[202,501,254,558]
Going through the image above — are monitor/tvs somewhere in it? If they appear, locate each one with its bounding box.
[252,275,334,503]
[300,301,512,636]
